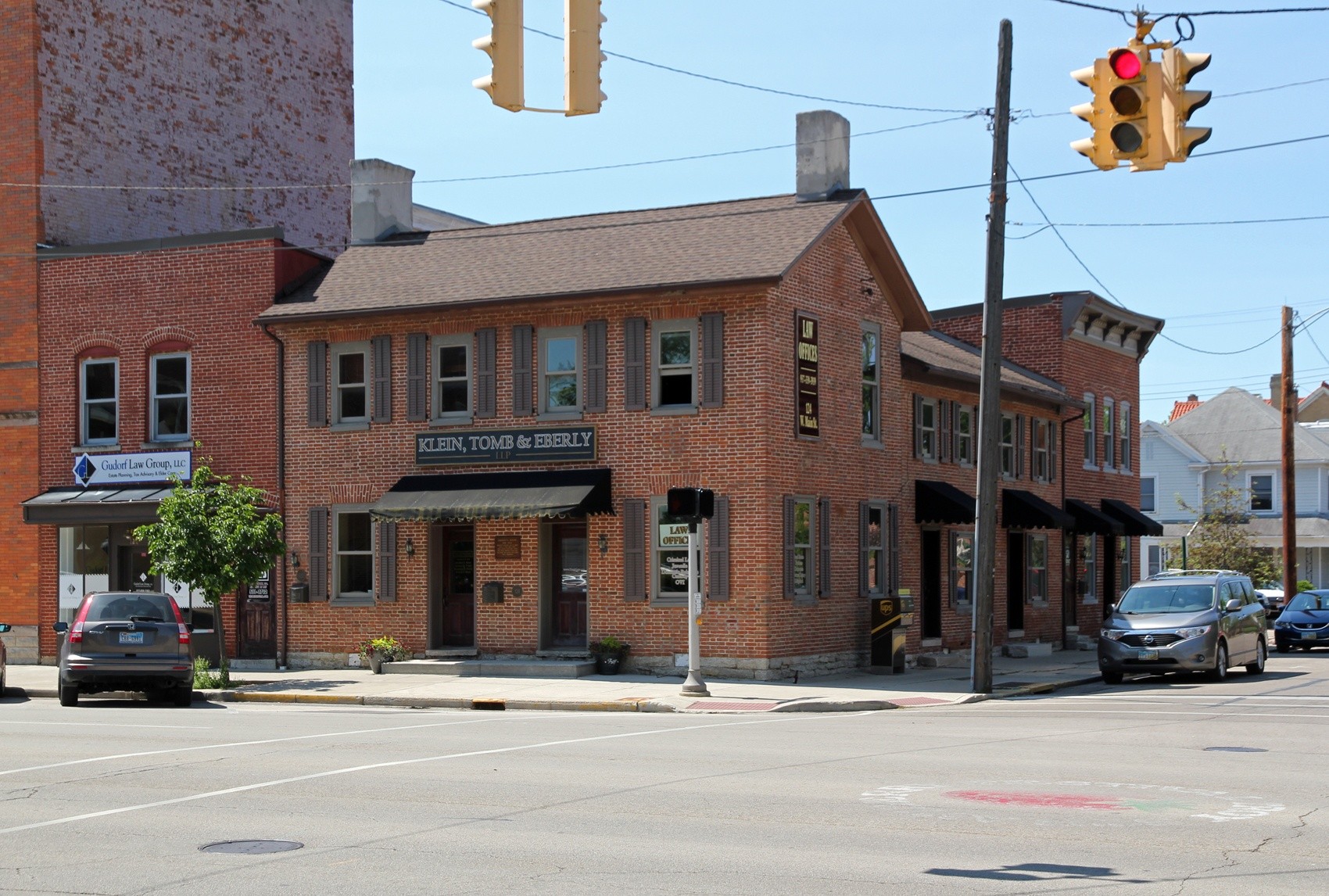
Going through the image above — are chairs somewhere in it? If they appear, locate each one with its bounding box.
[1145,590,1213,607]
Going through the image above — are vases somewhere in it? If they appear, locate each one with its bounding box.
[364,650,386,673]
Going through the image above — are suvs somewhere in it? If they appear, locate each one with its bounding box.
[1097,567,1270,686]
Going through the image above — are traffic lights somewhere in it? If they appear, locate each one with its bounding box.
[1161,47,1213,162]
[471,0,524,112]
[1068,58,1119,171]
[1108,42,1149,160]
[666,487,715,519]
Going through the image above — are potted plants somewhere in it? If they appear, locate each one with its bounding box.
[588,638,630,675]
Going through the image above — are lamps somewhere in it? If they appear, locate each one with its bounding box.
[405,539,416,560]
[1079,547,1089,563]
[862,288,874,296]
[290,550,300,574]
[1117,548,1126,564]
[597,534,608,556]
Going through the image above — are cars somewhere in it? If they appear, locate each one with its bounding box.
[52,589,195,706]
[0,622,12,695]
[1253,580,1285,608]
[1273,589,1329,652]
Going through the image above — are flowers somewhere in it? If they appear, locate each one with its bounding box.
[352,636,412,663]
